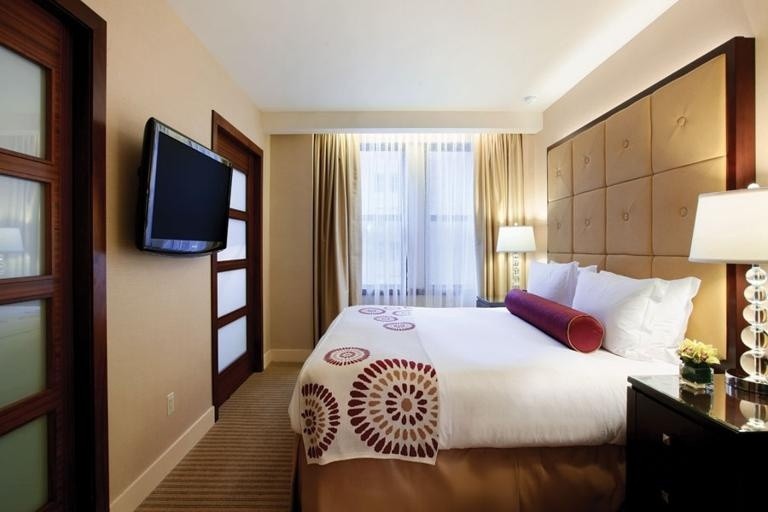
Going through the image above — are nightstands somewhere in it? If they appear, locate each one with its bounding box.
[625,371,768,512]
[476,295,506,308]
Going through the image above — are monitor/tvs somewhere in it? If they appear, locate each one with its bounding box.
[132,115,234,259]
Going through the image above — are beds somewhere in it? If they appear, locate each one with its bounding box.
[288,304,696,511]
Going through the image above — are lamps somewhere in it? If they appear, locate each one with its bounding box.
[686,183,768,397]
[494,221,537,296]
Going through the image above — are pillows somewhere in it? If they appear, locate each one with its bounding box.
[548,257,598,281]
[599,268,702,367]
[503,287,605,354]
[526,259,578,308]
[571,270,661,361]
